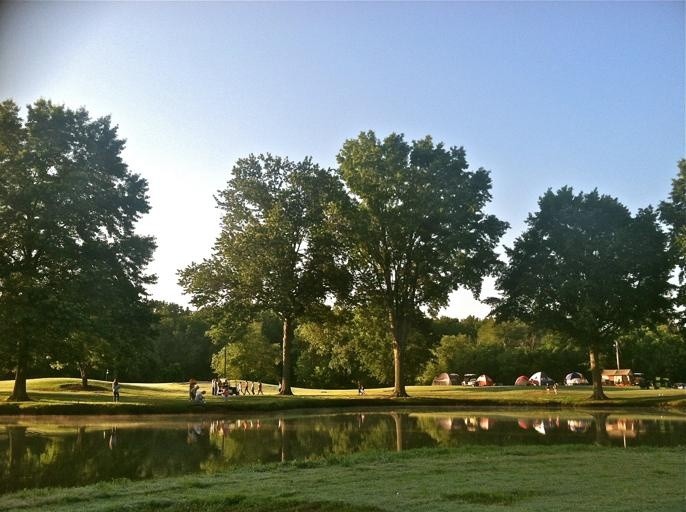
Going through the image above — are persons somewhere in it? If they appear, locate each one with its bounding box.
[192,417,281,443]
[109,426,119,450]
[357,381,364,396]
[112,378,120,402]
[189,376,282,402]
[545,382,559,395]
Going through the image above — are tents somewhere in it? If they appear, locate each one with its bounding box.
[440,416,592,436]
[432,371,589,387]
